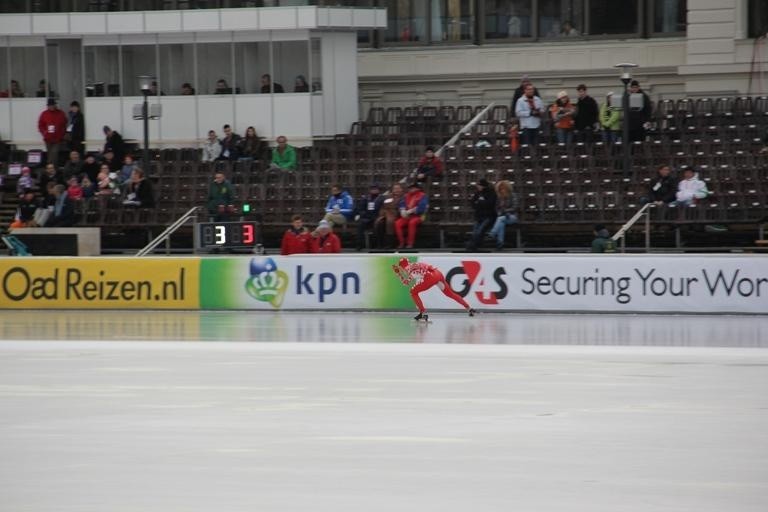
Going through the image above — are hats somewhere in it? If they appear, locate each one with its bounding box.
[631,80,639,86]
[557,91,569,98]
[317,219,330,229]
[521,73,530,82]
[46,98,57,107]
[477,179,488,186]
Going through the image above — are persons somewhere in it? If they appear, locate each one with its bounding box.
[559,21,578,38]
[392,258,476,320]
[508,12,522,38]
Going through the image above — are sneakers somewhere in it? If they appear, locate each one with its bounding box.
[414,312,428,320]
[468,307,475,317]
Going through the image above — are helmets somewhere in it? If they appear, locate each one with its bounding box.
[398,258,411,269]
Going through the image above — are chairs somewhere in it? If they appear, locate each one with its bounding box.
[333,96,768,250]
[3,140,332,253]
